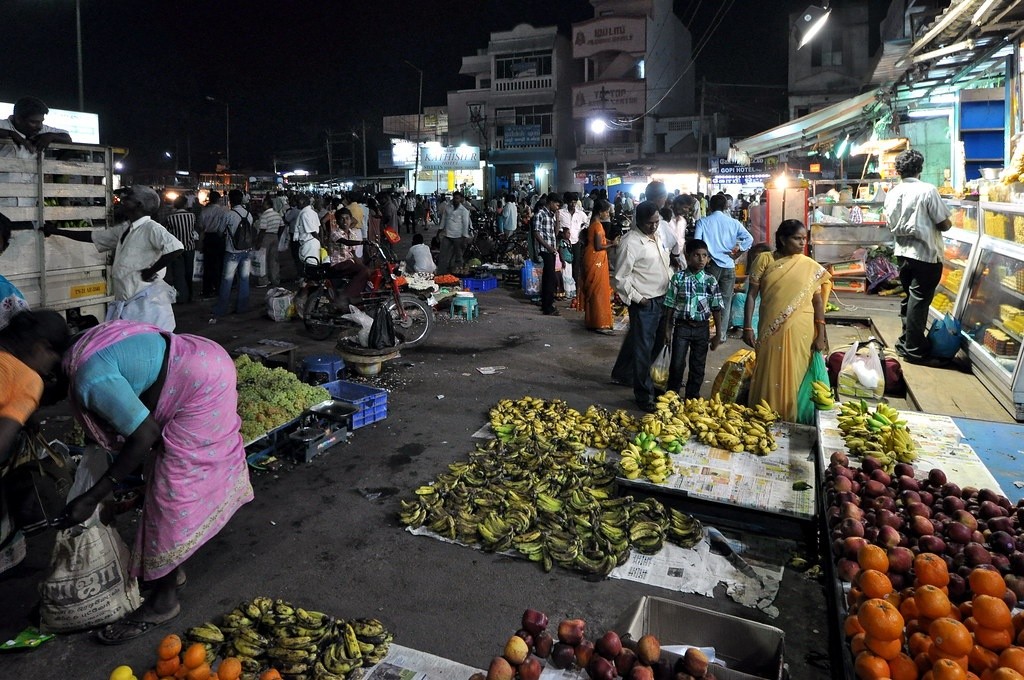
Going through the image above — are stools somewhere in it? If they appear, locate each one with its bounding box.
[230,338,300,373]
[450,297,479,320]
[300,355,347,382]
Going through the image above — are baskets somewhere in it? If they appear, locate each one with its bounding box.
[463,276,497,291]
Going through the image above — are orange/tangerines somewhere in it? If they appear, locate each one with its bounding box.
[144,634,283,680]
[845,545,1024,680]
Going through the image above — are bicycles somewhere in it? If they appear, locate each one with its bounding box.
[459,209,529,279]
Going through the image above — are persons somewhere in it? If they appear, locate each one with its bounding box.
[743,219,833,423]
[38,184,185,333]
[156,180,770,413]
[888,149,952,362]
[37,310,255,643]
[0,213,69,638]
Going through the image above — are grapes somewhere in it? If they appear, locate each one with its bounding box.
[232,354,332,444]
[64,424,88,448]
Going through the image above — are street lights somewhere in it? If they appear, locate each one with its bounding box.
[205,94,230,167]
[590,118,609,199]
[402,58,423,192]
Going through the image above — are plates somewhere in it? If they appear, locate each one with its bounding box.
[248,453,270,469]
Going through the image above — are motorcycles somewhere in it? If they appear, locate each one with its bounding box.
[302,235,434,349]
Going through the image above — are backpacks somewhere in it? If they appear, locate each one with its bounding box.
[227,209,253,250]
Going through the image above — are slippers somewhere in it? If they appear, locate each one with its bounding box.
[96,604,183,643]
[167,575,188,588]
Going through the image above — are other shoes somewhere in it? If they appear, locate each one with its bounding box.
[609,376,630,386]
[637,400,656,412]
[256,284,267,288]
[550,311,562,315]
[597,328,616,335]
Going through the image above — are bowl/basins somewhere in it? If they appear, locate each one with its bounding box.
[978,168,1003,179]
[356,362,382,376]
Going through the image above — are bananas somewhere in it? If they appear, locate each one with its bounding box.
[179,596,395,680]
[488,396,640,453]
[615,305,628,317]
[809,380,835,410]
[836,398,917,474]
[394,425,703,581]
[617,366,780,483]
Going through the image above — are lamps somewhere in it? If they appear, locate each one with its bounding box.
[794,0,833,51]
[833,114,882,159]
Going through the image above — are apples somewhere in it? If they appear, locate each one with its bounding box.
[570,298,577,308]
[825,451,1024,613]
[483,609,715,680]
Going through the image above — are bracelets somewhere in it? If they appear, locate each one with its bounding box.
[744,328,753,331]
[360,242,361,245]
[103,471,121,485]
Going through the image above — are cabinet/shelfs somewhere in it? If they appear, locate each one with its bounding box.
[927,178,1024,421]
[812,179,898,264]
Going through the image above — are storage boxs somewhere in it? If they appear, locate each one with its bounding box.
[615,595,785,680]
[818,249,868,292]
[322,380,390,430]
[463,276,497,292]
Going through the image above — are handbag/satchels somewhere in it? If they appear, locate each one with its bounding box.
[555,251,562,272]
[278,225,290,252]
[248,250,266,276]
[797,350,830,426]
[837,340,884,400]
[651,345,671,390]
[926,312,962,360]
[5,429,81,533]
[368,304,395,349]
[558,238,574,263]
[342,304,374,347]
[39,522,144,631]
